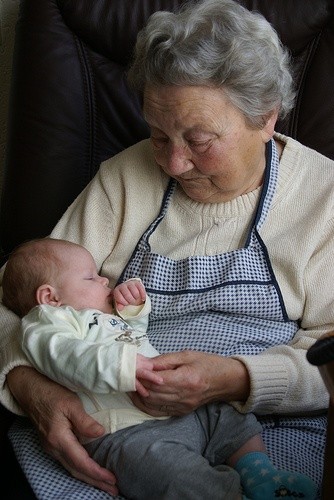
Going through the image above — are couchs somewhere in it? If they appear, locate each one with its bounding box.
[0,0,334,500]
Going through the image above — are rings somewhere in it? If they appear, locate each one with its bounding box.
[158,404,168,412]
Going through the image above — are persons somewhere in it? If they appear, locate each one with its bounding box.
[0,0,334,500]
[2,238,318,500]
